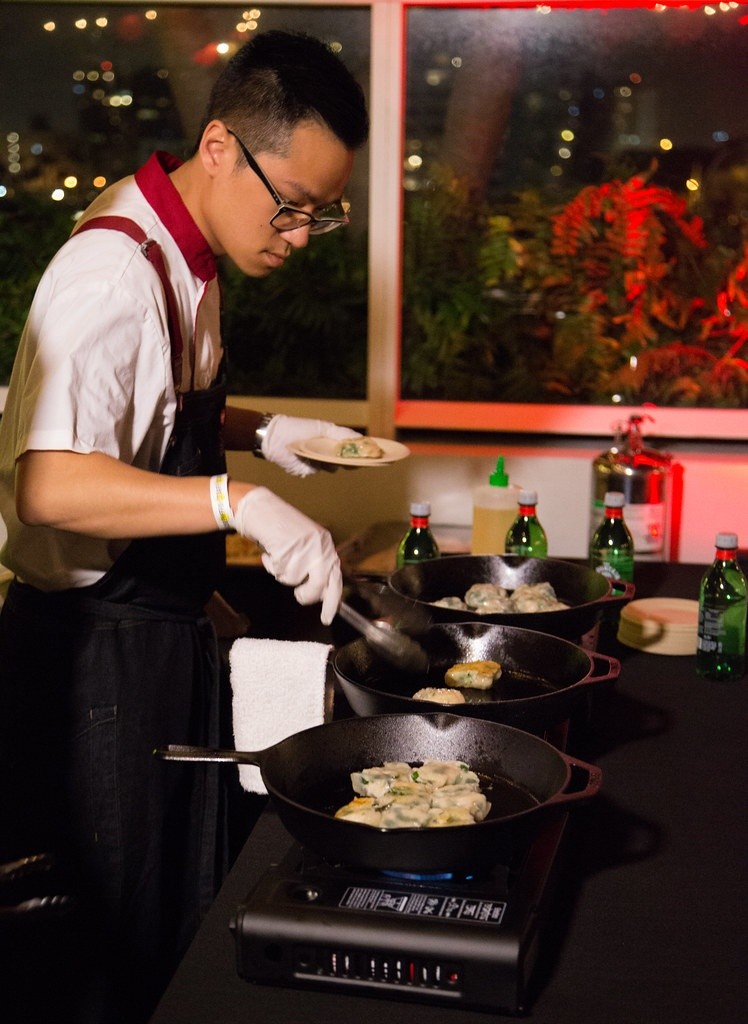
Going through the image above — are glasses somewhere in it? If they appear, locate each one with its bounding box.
[225,127,350,236]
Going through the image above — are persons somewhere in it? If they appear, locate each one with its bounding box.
[1,25,365,1024]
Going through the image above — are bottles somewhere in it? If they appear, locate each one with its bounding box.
[397,502,440,568]
[695,533,748,681]
[472,455,524,554]
[505,491,547,559]
[589,492,634,595]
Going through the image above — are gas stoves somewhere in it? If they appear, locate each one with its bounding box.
[228,840,550,1007]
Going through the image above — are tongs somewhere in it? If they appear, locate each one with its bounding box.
[258,541,429,674]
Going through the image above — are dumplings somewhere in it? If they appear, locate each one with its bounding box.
[338,438,380,457]
[443,660,501,689]
[334,759,490,828]
[413,687,465,704]
[429,583,567,614]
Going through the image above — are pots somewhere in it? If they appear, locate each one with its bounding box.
[328,623,619,717]
[378,553,635,638]
[151,713,601,863]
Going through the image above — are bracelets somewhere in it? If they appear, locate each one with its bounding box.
[208,472,237,534]
[251,410,277,461]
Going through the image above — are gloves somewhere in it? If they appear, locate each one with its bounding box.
[259,412,368,480]
[231,486,346,627]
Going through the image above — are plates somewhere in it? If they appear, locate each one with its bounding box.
[285,435,409,467]
